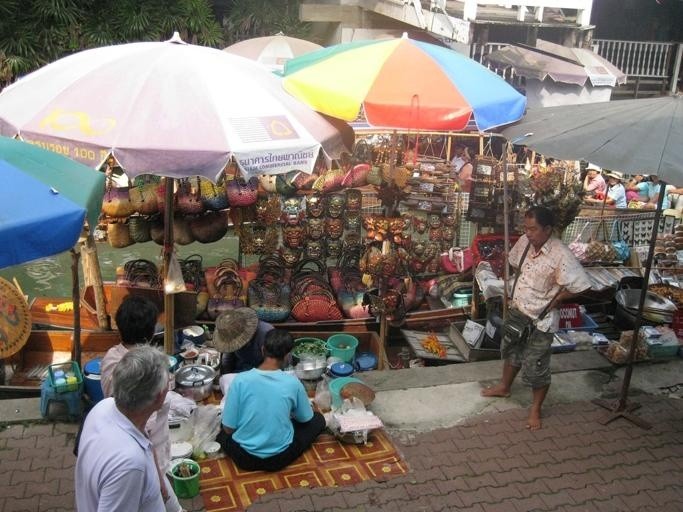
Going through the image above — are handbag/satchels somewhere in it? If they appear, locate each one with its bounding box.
[224,161,411,207]
[567,241,630,264]
[93,175,228,245]
[407,150,459,214]
[124,244,424,326]
[467,156,583,233]
[440,246,473,274]
[502,307,536,346]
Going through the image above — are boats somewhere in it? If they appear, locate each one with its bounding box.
[0,257,485,395]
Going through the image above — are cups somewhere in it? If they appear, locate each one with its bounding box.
[170,462,198,500]
[54,369,77,393]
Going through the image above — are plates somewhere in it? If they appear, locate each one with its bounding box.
[179,347,200,359]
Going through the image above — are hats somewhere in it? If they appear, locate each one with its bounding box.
[585,163,601,173]
[212,306,258,354]
[606,171,624,180]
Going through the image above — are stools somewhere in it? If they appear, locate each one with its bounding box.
[38,376,83,416]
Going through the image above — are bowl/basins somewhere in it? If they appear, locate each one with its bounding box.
[168,438,193,459]
[291,336,329,361]
[294,362,326,380]
[203,442,221,457]
[168,354,177,370]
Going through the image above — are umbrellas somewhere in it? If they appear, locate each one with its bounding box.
[0,136,107,267]
[0,31,356,395]
[500,93,683,412]
[480,40,627,88]
[218,31,330,77]
[273,31,528,256]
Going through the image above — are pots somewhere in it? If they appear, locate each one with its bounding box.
[614,275,681,325]
[167,408,196,444]
[174,364,216,401]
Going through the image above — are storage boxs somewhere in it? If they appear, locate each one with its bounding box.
[638,327,681,358]
[471,233,521,265]
[451,290,471,306]
[554,309,602,333]
[448,319,501,361]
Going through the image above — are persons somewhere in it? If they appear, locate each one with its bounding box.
[216,329,327,474]
[451,143,466,173]
[99,295,170,478]
[74,344,186,512]
[457,147,475,181]
[211,306,294,374]
[583,165,682,215]
[480,206,592,431]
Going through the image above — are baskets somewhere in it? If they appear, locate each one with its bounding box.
[48,361,84,395]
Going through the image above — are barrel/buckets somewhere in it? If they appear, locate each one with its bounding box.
[168,356,177,391]
[165,460,201,500]
[328,376,368,409]
[453,292,472,308]
[82,356,103,404]
